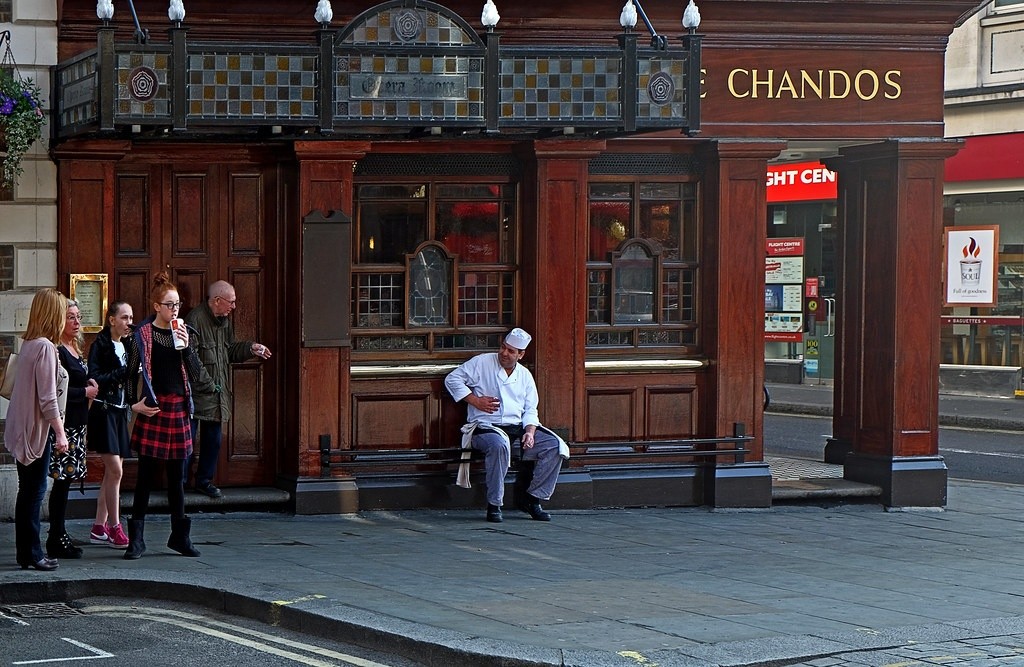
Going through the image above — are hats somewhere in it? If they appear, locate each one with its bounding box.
[504,328,532,349]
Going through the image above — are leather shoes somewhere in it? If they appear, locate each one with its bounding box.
[487,502,503,522]
[195,482,221,497]
[519,495,551,521]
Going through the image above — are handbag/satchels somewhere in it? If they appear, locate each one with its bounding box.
[93,382,135,408]
[0,352,18,400]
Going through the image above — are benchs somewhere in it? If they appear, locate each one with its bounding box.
[320,430,530,499]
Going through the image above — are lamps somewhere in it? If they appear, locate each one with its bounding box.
[314,0,333,29]
[96,0,114,21]
[618,0,638,34]
[168,0,186,26]
[682,0,701,33]
[480,0,501,34]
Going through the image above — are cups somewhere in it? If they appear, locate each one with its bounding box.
[169,318,185,350]
[960,259,982,286]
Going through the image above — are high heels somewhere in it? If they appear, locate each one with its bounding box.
[21,558,59,570]
[46,533,83,559]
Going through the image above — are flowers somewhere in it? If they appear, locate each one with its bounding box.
[0,61,47,194]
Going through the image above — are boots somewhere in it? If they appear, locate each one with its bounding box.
[167,515,201,557]
[124,517,146,558]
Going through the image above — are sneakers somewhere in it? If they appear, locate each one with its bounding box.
[90,522,111,544]
[108,522,129,548]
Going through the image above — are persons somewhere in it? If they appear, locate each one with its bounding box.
[124,272,201,559]
[4,288,69,571]
[445,328,569,522]
[87,301,131,549]
[186,280,273,498]
[47,300,99,559]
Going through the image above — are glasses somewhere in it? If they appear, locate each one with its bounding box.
[67,316,82,320]
[159,302,183,310]
[214,296,236,305]
